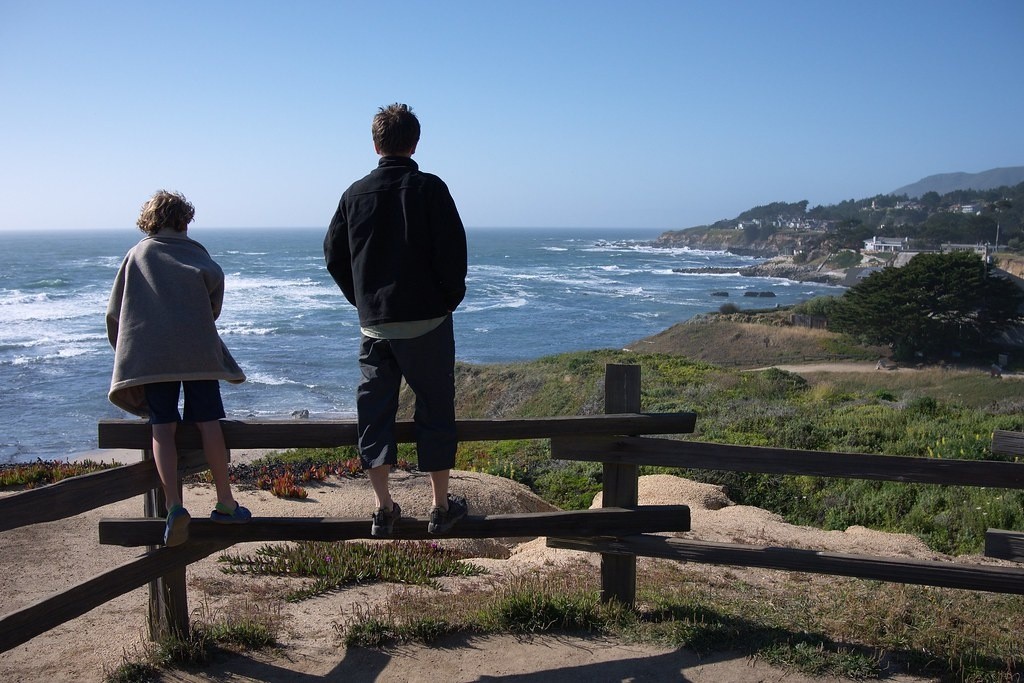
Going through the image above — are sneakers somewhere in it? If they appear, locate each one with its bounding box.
[428,494,468,535]
[370,503,401,537]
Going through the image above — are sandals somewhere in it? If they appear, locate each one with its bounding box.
[210,500,252,525]
[163,503,191,547]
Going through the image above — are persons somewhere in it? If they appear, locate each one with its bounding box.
[104,189,252,547]
[322,103,469,537]
